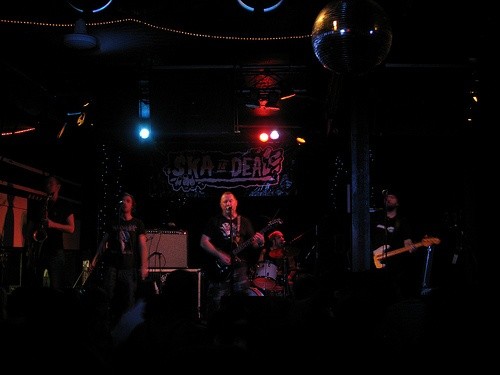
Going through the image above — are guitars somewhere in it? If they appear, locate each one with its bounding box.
[210,216,283,275]
[373,236,441,268]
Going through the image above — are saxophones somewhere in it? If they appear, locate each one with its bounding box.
[32,193,55,243]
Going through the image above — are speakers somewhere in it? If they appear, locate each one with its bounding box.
[140,230,188,270]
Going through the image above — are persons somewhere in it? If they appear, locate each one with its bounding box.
[0,255,500,375]
[92,192,145,295]
[200,192,265,297]
[41,176,76,250]
[372,192,417,296]
[257,229,297,297]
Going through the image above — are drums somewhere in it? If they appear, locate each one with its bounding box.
[253,260,279,289]
[248,287,264,297]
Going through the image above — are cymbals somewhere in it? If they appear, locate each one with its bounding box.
[269,247,298,258]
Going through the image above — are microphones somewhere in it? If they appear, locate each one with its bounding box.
[382,190,388,195]
[226,206,231,214]
[119,201,123,208]
[162,222,175,226]
